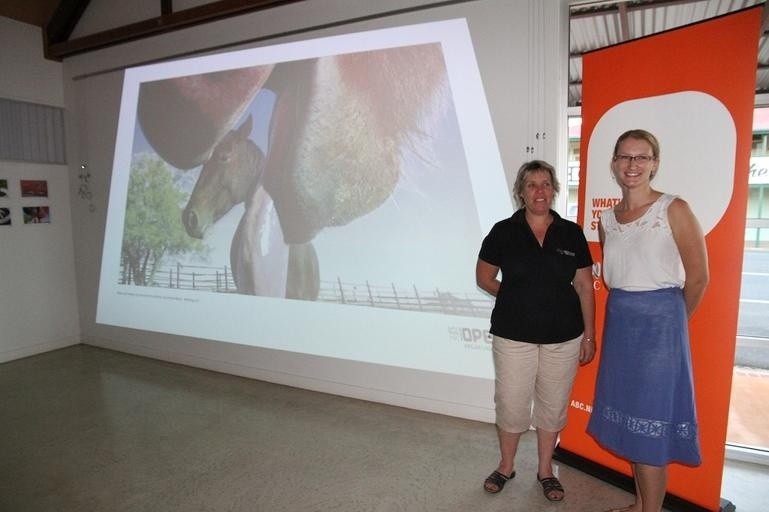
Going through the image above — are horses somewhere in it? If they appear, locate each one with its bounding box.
[176,110,322,303]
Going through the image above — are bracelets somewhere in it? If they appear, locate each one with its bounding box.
[583,337,593,343]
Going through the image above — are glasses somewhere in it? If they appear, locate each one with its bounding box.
[614,156,656,164]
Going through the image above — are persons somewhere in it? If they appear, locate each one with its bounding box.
[476,160,597,502]
[586,129,709,512]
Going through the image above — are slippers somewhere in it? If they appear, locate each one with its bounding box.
[484,471,515,493]
[537,472,564,501]
